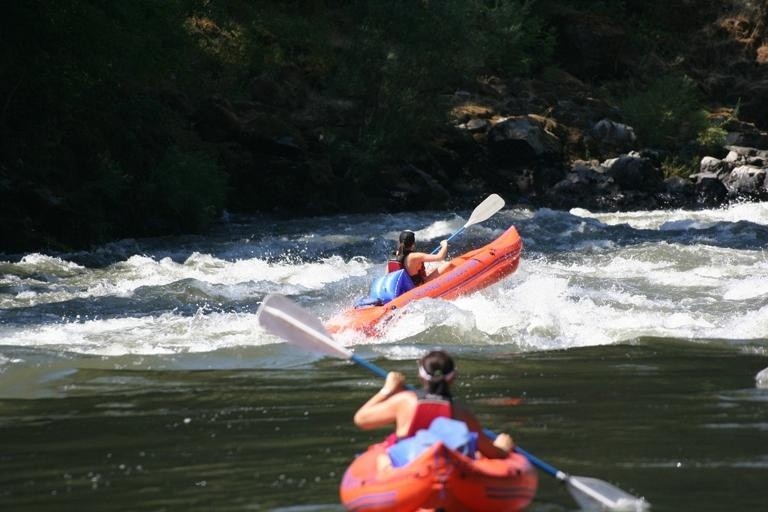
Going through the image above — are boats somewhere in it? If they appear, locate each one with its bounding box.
[322,225,524,341]
[338,431,541,511]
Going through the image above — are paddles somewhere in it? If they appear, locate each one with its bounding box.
[430,194,505,256]
[256,296,650,512]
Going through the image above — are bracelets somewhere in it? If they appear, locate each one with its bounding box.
[380,388,391,396]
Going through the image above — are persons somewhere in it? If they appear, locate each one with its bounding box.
[353,351,513,461]
[385,231,448,285]
